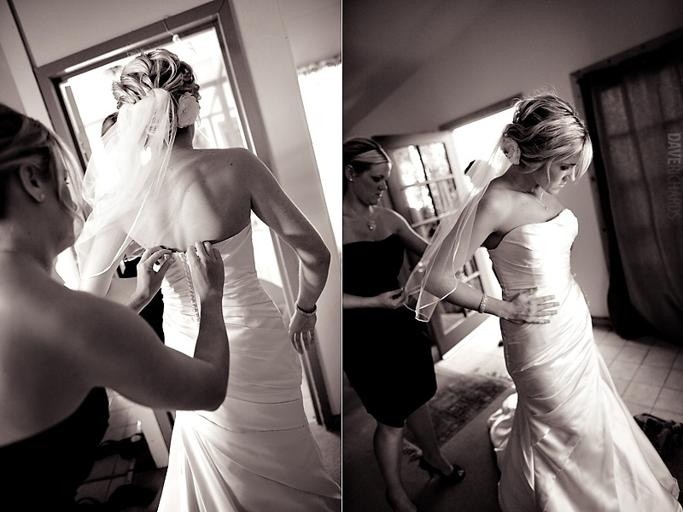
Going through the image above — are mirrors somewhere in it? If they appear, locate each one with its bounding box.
[54,24,319,438]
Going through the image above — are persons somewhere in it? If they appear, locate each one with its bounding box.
[463,160,505,347]
[344,136,467,511]
[405,94,682,511]
[0,103,229,512]
[73,47,341,511]
[102,113,168,348]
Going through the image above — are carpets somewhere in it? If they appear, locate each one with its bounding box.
[367,364,517,457]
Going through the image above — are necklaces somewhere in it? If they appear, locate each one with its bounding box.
[345,202,377,232]
[507,172,551,212]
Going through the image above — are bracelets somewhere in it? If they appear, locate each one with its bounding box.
[478,294,488,314]
[294,303,316,318]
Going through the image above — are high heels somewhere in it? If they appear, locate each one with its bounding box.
[418,455,465,484]
